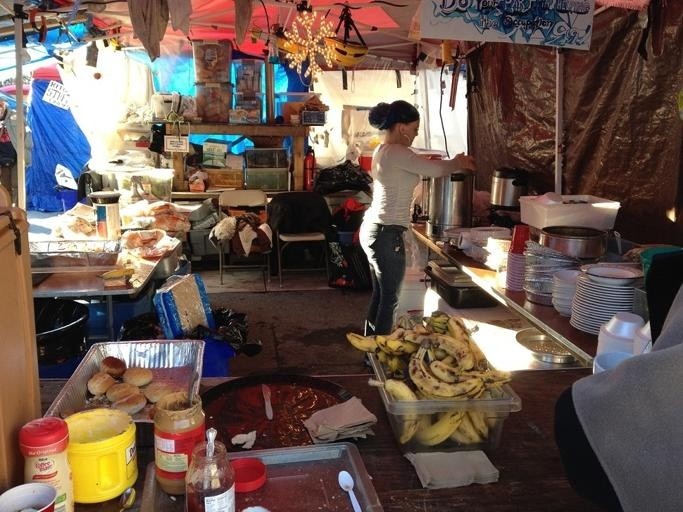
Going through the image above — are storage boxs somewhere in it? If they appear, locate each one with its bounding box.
[275,91,320,124]
[114,167,175,204]
[192,81,233,124]
[358,149,445,173]
[231,58,265,95]
[201,138,289,193]
[187,37,232,84]
[234,94,263,126]
[367,333,522,453]
[69,280,157,341]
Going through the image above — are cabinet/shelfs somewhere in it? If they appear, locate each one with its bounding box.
[0,211,44,493]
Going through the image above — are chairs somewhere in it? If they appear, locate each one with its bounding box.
[211,189,272,284]
[268,191,332,288]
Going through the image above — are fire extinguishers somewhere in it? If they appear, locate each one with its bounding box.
[305,147,317,190]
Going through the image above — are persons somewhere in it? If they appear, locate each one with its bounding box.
[0,97,19,207]
[354,97,478,370]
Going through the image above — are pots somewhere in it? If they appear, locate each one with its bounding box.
[426,168,474,242]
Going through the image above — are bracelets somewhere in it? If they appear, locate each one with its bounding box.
[457,156,467,170]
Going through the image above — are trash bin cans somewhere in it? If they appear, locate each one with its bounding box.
[54,178,88,212]
[120,308,233,377]
[154,260,189,291]
[34,299,89,378]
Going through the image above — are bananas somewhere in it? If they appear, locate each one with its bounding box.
[346,309,513,447]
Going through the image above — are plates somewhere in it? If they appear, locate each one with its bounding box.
[523,239,648,357]
[515,326,578,365]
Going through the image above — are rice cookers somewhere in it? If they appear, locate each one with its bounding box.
[489,167,526,212]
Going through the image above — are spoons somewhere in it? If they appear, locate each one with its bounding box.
[336,469,361,512]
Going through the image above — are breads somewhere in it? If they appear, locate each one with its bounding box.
[86,356,172,414]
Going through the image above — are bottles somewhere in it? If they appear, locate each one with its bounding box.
[19,417,74,512]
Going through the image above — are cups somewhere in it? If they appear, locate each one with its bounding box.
[0,482,58,512]
[504,225,527,293]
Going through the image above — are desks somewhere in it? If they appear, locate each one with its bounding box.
[407,223,599,367]
[37,366,605,511]
[30,230,175,341]
